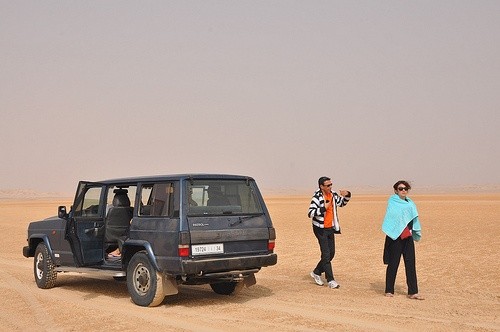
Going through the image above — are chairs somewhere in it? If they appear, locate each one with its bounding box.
[105,194,134,242]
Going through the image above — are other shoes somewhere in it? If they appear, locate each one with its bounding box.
[108,247,122,257]
[408,293,425,300]
[386,292,393,297]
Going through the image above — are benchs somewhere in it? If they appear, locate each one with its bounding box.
[190,205,241,212]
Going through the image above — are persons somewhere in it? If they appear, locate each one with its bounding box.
[381,180,424,300]
[307,177,351,288]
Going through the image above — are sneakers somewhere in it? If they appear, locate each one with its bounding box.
[310,271,324,286]
[327,280,340,288]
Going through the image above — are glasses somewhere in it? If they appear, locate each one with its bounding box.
[397,187,408,191]
[324,183,333,187]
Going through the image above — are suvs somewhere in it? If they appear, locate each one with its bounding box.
[24,174,279,306]
